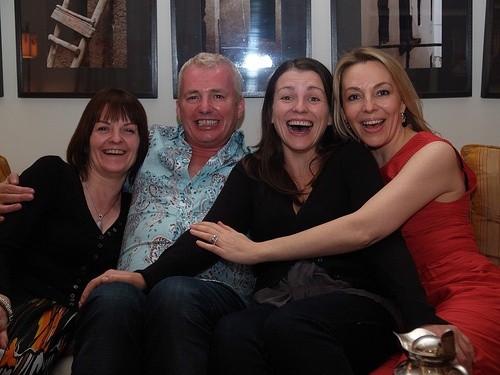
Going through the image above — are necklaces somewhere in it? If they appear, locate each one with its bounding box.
[85,179,118,232]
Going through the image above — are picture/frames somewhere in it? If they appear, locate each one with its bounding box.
[13,0,158,100]
[330,0,473,99]
[480,0,500,100]
[170,0,312,98]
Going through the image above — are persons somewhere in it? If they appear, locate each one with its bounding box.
[0,47,500,375]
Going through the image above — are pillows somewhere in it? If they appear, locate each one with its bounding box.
[460,145,499,267]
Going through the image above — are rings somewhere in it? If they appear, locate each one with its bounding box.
[210,235,219,246]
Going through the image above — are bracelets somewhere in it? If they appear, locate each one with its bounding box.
[0,296,14,329]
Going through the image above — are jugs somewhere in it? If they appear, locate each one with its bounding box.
[393,328,468,375]
